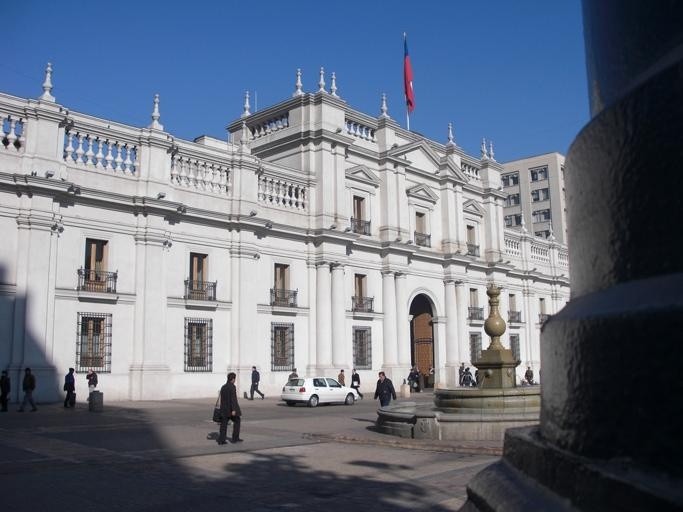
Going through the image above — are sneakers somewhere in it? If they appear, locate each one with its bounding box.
[30,408,37,412]
[249,398,253,400]
[64,405,69,407]
[262,394,264,400]
[1,408,8,412]
[17,409,24,412]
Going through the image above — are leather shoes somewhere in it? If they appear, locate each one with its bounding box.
[232,439,243,442]
[218,441,227,444]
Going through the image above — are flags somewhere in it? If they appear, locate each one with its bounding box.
[400,33,415,112]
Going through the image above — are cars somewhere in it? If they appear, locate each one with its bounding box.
[280,376,359,408]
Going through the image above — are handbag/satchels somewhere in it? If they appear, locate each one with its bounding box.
[213,408,221,422]
[70,393,76,404]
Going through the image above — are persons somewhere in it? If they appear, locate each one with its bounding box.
[217,372,244,445]
[84,367,97,400]
[349,369,362,400]
[0,369,13,412]
[17,367,38,413]
[287,368,298,382]
[523,366,533,385]
[337,370,345,386]
[62,367,75,408]
[247,366,263,401]
[408,361,478,394]
[373,371,397,409]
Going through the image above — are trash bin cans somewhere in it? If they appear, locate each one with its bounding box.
[89,391,103,411]
